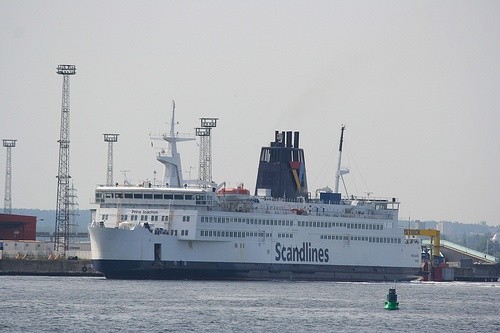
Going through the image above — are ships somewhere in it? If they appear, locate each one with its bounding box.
[86,99,428,282]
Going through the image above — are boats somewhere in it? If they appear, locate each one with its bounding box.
[382,287,400,311]
